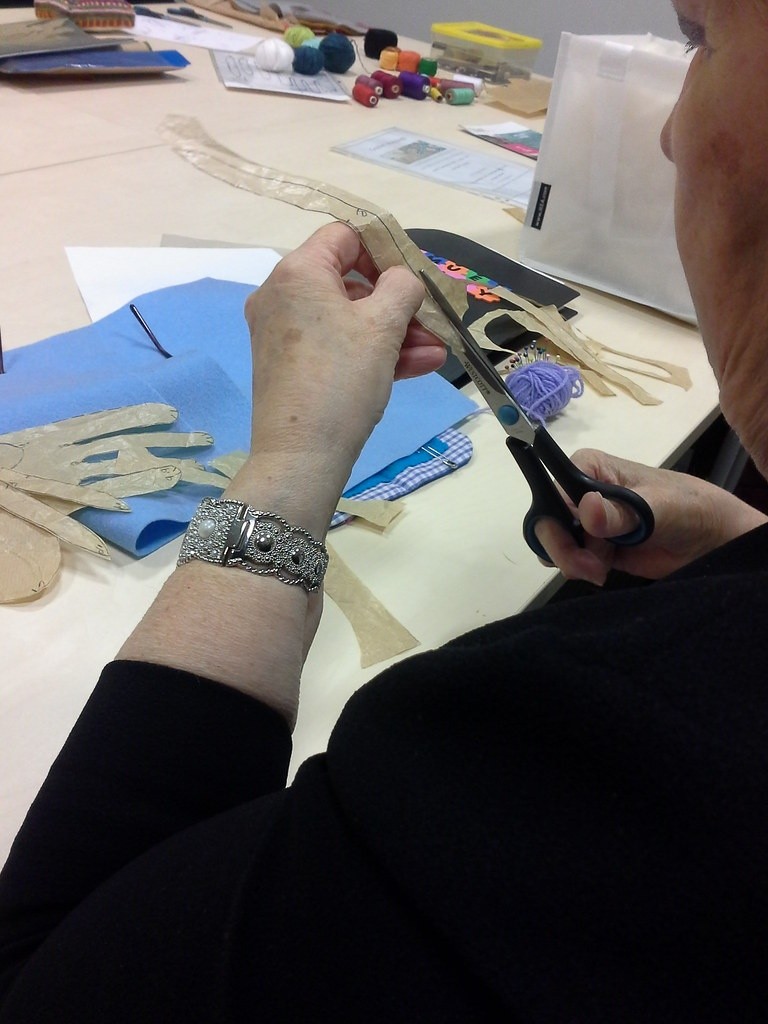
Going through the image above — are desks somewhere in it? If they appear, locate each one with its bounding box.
[0,5,722,874]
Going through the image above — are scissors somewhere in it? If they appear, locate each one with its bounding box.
[419,268,656,567]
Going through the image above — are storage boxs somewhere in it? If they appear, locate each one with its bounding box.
[430,22,543,84]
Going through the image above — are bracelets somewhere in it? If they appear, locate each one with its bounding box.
[173,495,329,595]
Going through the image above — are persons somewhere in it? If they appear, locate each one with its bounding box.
[1,4,768,1024]
